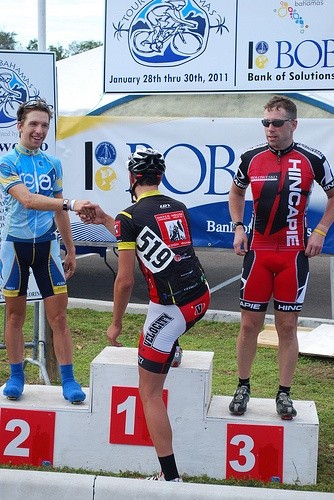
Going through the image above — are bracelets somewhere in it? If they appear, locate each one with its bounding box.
[70,200,75,210]
[314,229,326,236]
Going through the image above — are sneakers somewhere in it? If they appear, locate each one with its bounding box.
[275,392,297,421]
[228,386,251,413]
[145,469,183,483]
[170,346,182,367]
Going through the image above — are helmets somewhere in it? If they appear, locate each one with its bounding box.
[127,148,166,178]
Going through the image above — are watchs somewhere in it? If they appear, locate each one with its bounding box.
[233,222,245,231]
[63,199,68,211]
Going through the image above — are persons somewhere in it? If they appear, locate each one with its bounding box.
[0,99,96,405]
[228,96,334,420]
[75,148,211,483]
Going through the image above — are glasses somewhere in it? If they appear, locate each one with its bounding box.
[261,118,292,127]
[24,100,49,108]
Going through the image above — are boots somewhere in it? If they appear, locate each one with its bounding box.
[4,362,25,400]
[60,364,86,404]
